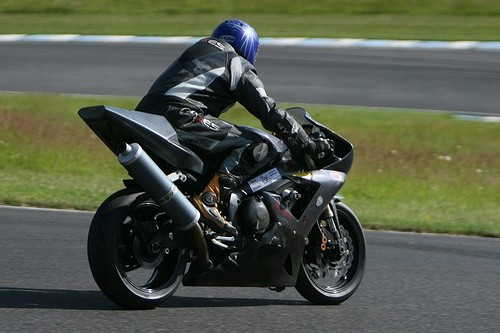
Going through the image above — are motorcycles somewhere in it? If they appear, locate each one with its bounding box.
[77,103,367,309]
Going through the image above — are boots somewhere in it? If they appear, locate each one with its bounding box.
[194,176,239,235]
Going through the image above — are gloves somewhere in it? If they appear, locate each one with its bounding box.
[315,137,335,153]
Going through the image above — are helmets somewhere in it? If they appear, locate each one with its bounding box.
[212,20,258,65]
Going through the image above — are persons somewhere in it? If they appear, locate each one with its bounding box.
[134,19,330,236]
